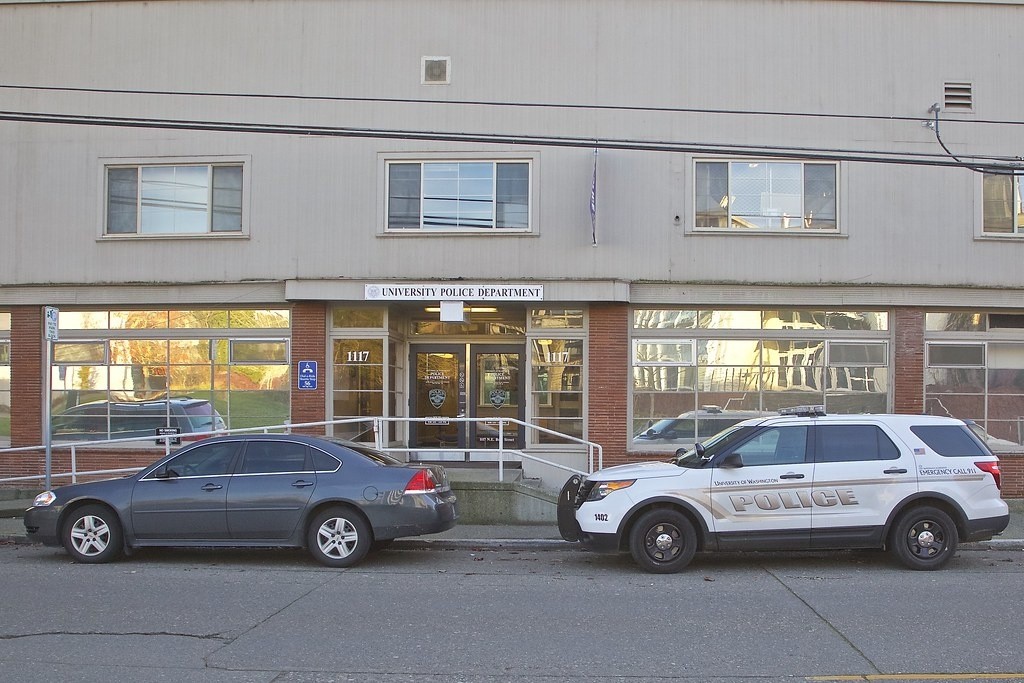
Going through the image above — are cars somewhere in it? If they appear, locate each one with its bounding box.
[23,431,460,569]
[346,419,507,450]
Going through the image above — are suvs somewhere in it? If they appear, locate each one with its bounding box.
[555,408,1011,574]
[631,402,782,459]
[50,394,234,445]
